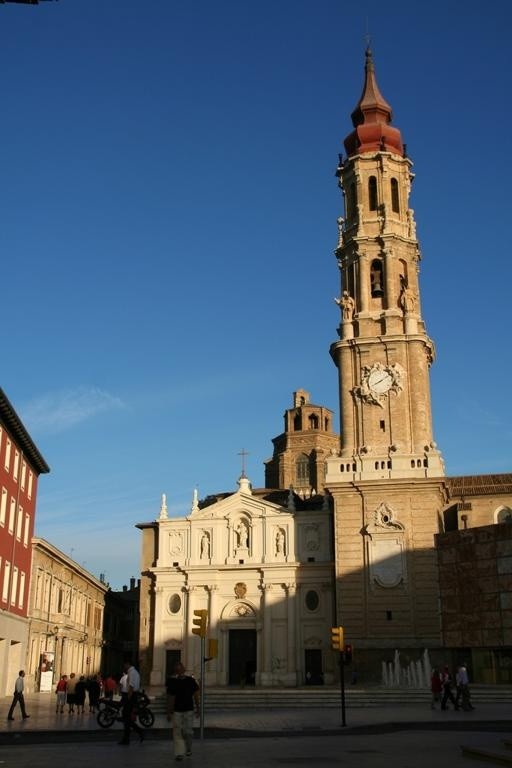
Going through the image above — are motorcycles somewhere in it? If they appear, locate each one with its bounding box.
[96,688,155,727]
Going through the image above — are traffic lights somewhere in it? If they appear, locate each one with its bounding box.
[345,639,353,665]
[192,609,207,637]
[330,625,345,652]
[208,639,219,659]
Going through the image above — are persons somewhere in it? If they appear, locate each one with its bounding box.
[166,661,201,761]
[8,669,31,720]
[55,672,118,715]
[430,660,476,712]
[119,669,129,698]
[165,672,176,713]
[116,661,147,746]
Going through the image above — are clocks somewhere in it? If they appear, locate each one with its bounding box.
[368,371,393,395]
